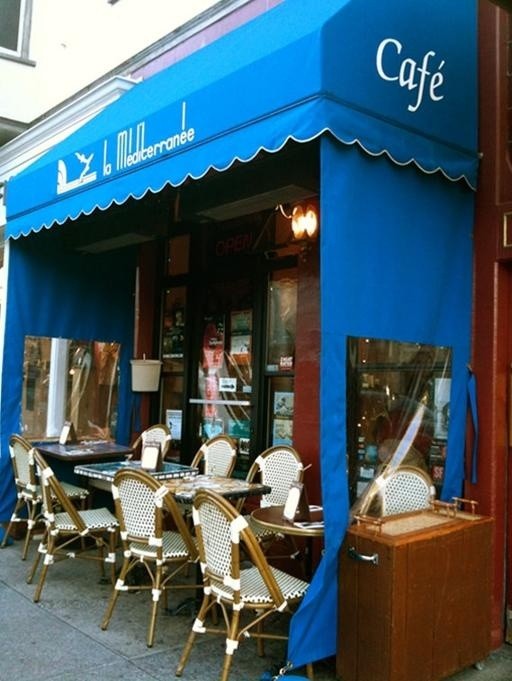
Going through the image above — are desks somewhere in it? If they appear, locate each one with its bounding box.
[156,470,273,522]
[35,433,137,497]
[71,454,200,496]
[247,500,332,541]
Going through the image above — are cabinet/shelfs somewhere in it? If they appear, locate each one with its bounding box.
[336,505,495,681]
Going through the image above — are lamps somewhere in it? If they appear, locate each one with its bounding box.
[273,201,319,243]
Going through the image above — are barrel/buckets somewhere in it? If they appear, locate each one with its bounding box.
[131,358,164,393]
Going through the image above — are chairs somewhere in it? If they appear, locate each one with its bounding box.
[2,431,92,560]
[97,466,221,653]
[232,442,311,549]
[30,449,121,609]
[189,432,239,483]
[127,423,174,462]
[353,463,431,526]
[172,485,316,681]
